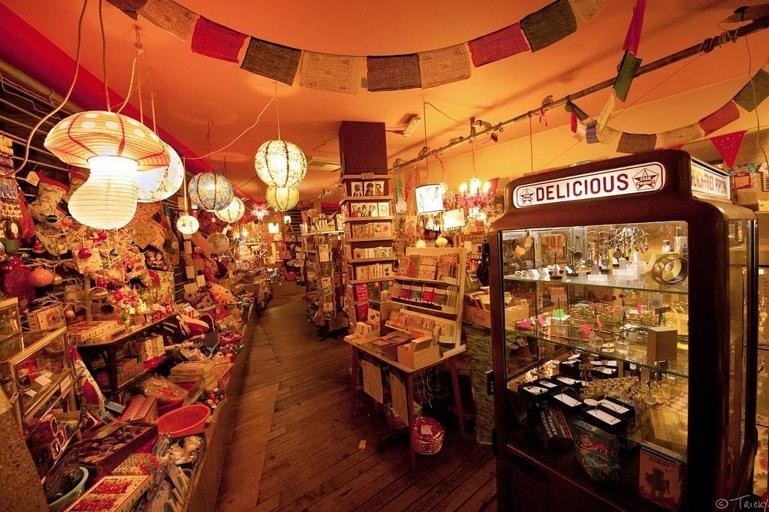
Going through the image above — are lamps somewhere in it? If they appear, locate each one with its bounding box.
[127,50,187,204]
[410,97,469,233]
[253,87,307,212]
[187,105,234,212]
[45,2,170,231]
[214,145,245,224]
[176,160,201,237]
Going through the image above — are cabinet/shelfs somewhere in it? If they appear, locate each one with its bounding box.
[306,147,769,511]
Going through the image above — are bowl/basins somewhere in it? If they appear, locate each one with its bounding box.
[48,466,90,512]
[154,404,211,438]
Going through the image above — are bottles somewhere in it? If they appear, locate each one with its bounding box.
[477,242,491,286]
